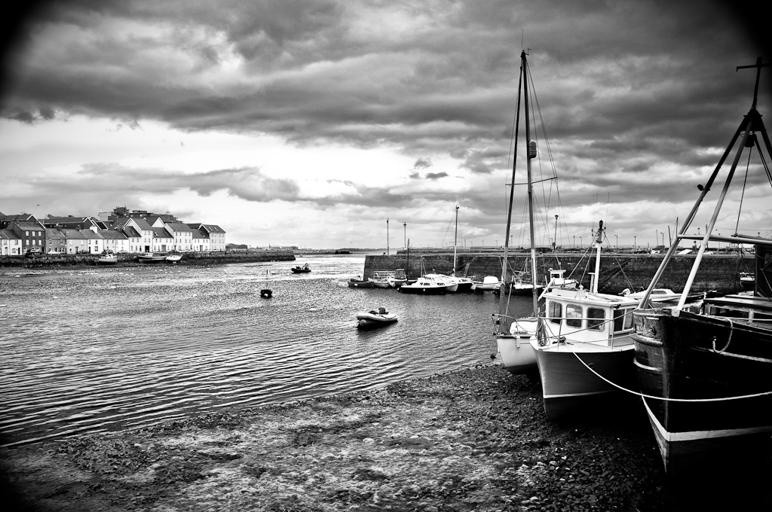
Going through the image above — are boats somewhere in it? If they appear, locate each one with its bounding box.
[292,268,311,272]
[355,307,397,330]
[138,255,183,264]
[98,253,117,265]
[348,207,544,294]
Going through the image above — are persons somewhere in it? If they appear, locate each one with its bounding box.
[379,307,388,314]
